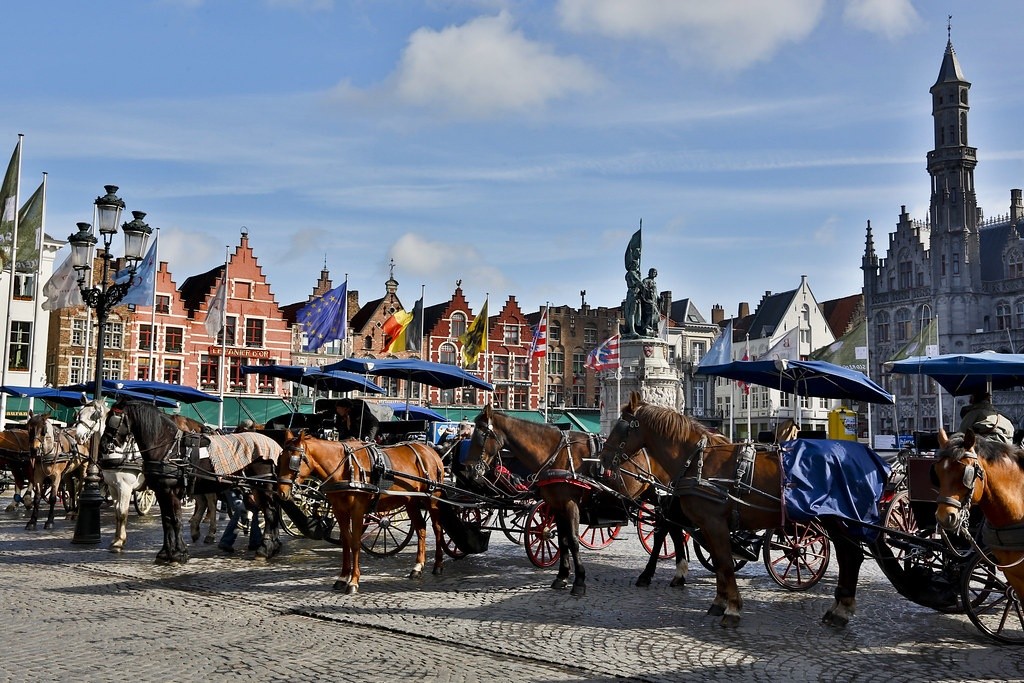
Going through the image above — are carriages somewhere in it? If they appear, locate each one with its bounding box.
[0,350,1023,650]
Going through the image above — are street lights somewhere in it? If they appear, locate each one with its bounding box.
[538,389,566,426]
[839,402,860,441]
[68,185,152,543]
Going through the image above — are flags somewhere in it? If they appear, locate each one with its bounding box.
[810,321,867,372]
[380,298,422,353]
[205,270,226,337]
[0,141,20,274]
[458,299,487,367]
[759,326,798,361]
[737,354,751,395]
[529,309,547,357]
[4,182,43,274]
[111,237,157,306]
[296,281,346,351]
[583,334,618,372]
[889,316,939,360]
[698,321,731,366]
[41,225,94,310]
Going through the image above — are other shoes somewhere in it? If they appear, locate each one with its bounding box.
[218,544,234,553]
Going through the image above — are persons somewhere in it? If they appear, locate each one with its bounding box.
[324,399,377,439]
[625,261,660,336]
[959,392,1014,445]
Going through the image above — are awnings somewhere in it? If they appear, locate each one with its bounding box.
[0,397,62,417]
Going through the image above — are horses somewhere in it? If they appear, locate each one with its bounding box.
[933,426,1024,602]
[100,394,286,567]
[0,430,33,514]
[275,426,444,593]
[75,394,219,555]
[463,402,688,596]
[597,390,891,633]
[25,409,90,531]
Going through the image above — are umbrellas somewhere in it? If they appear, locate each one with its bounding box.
[0,379,223,408]
[695,360,895,423]
[239,357,493,422]
[884,350,1024,406]
